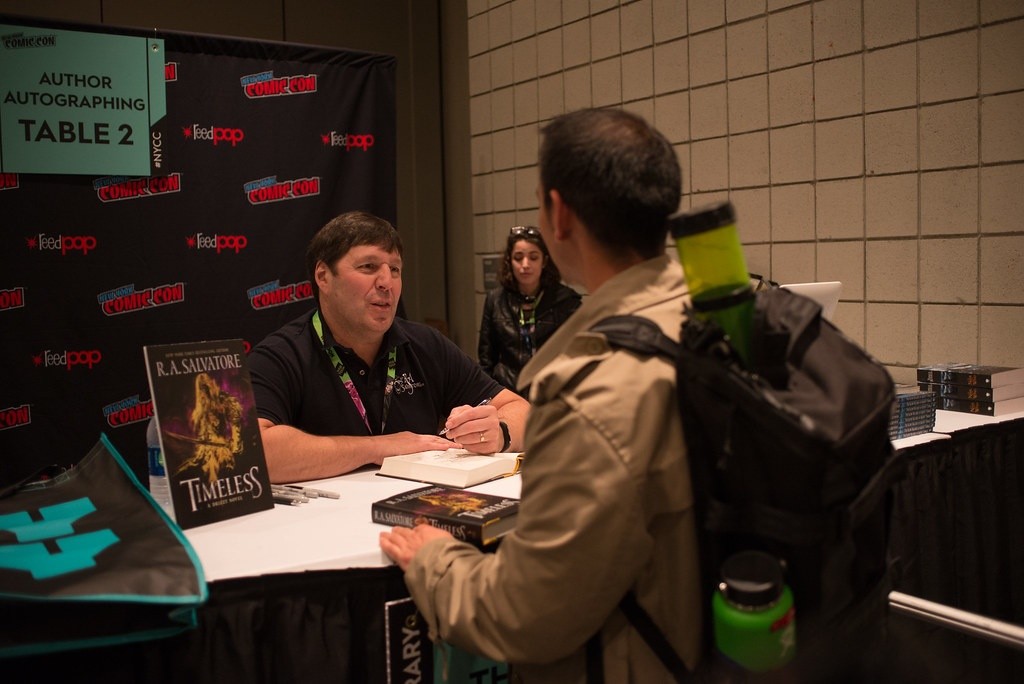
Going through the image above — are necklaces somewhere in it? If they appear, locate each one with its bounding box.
[517,287,541,302]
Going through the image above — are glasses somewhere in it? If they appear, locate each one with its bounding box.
[510,226,540,236]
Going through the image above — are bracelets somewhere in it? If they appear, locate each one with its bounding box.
[499,422,511,454]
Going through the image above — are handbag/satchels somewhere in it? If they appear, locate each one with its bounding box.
[0,431,208,657]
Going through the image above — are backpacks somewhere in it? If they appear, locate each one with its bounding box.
[587,273,904,684]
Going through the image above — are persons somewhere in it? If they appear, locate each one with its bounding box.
[477,226,581,403]
[380,108,767,684]
[243,215,532,484]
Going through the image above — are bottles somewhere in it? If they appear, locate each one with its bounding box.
[712,551,796,672]
[147,416,174,516]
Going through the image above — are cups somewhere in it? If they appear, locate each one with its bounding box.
[668,202,756,366]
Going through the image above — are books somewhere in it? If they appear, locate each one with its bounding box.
[374,444,526,547]
[888,362,1024,439]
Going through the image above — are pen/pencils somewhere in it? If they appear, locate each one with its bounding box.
[270,484,340,507]
[438,397,491,436]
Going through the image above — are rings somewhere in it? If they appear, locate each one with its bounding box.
[479,432,484,442]
